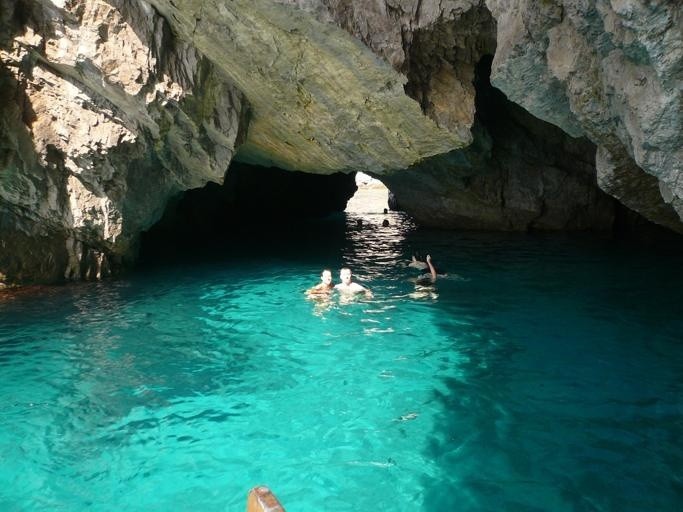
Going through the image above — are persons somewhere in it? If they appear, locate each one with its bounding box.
[305,268,336,305]
[335,268,374,306]
[407,253,447,299]
